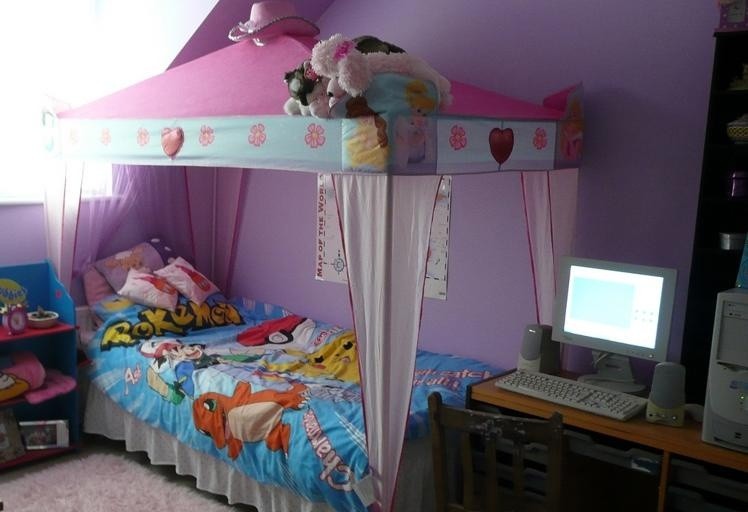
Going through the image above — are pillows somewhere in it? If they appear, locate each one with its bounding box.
[88,294,134,321]
[116,268,179,312]
[90,236,177,294]
[154,256,222,303]
[82,264,118,331]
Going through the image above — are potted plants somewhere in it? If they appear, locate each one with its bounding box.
[27,304,60,329]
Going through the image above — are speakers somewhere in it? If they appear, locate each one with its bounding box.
[645,361,686,428]
[517,324,557,374]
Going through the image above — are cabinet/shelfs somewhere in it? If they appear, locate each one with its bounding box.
[678,32,748,404]
[0,259,80,470]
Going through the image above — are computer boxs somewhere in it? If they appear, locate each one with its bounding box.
[701,288,748,454]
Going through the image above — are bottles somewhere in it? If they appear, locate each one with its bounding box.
[714,167,745,291]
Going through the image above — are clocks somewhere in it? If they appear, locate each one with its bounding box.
[0,303,29,335]
[712,0,748,32]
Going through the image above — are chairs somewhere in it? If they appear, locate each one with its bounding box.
[427,390,615,511]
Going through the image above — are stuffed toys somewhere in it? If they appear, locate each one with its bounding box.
[280,34,453,119]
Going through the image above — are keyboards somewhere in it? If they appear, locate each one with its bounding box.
[495,367,648,421]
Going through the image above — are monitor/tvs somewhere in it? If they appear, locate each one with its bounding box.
[553,254,680,392]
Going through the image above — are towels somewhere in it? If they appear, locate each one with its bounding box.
[24,370,77,405]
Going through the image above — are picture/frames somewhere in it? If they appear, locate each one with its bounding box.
[19,419,71,450]
[0,406,26,465]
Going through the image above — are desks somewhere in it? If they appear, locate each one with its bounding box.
[464,364,747,511]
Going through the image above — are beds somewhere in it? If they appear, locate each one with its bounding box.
[72,291,517,512]
